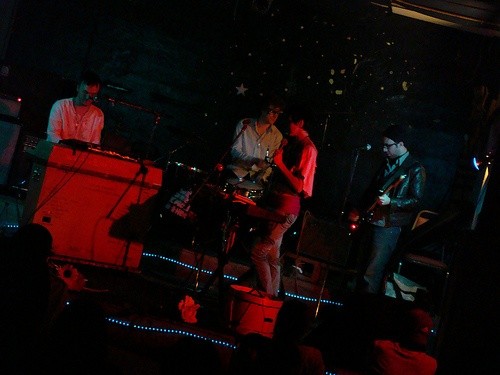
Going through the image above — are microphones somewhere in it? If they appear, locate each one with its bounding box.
[240,121,248,133]
[354,144,372,151]
[267,138,288,166]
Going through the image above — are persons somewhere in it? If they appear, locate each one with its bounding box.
[47,72,105,149]
[245,107,318,296]
[346,127,422,303]
[6,223,70,320]
[223,101,285,183]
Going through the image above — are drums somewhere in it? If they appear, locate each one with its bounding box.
[224,179,265,201]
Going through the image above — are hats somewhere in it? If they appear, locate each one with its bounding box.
[408,309,435,345]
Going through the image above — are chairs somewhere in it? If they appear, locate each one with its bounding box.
[395,211,455,299]
[274,210,339,296]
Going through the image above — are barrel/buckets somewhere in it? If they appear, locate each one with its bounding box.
[223,285,284,339]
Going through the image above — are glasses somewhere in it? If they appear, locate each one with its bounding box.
[382,142,398,147]
[266,108,280,115]
